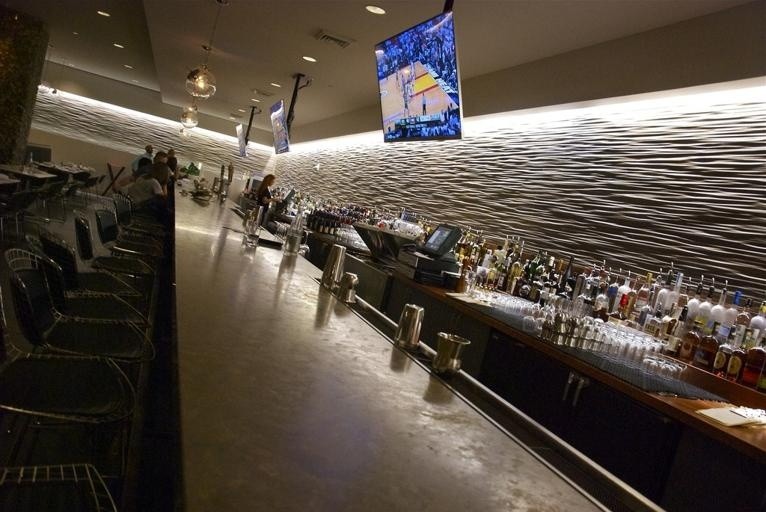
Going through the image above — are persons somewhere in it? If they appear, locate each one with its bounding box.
[258,174,281,226]
[132,145,178,230]
[375,11,460,140]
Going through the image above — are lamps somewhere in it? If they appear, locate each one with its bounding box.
[181,95,199,128]
[185,0,231,97]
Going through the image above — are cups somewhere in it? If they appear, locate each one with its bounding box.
[321,242,346,293]
[393,303,425,351]
[430,331,472,378]
[337,271,359,304]
[240,219,263,248]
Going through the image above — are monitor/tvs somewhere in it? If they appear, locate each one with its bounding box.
[373,10,465,142]
[235,123,248,157]
[269,98,291,155]
[422,223,463,257]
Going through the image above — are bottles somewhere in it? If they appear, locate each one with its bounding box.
[455,229,766,395]
[273,185,433,261]
